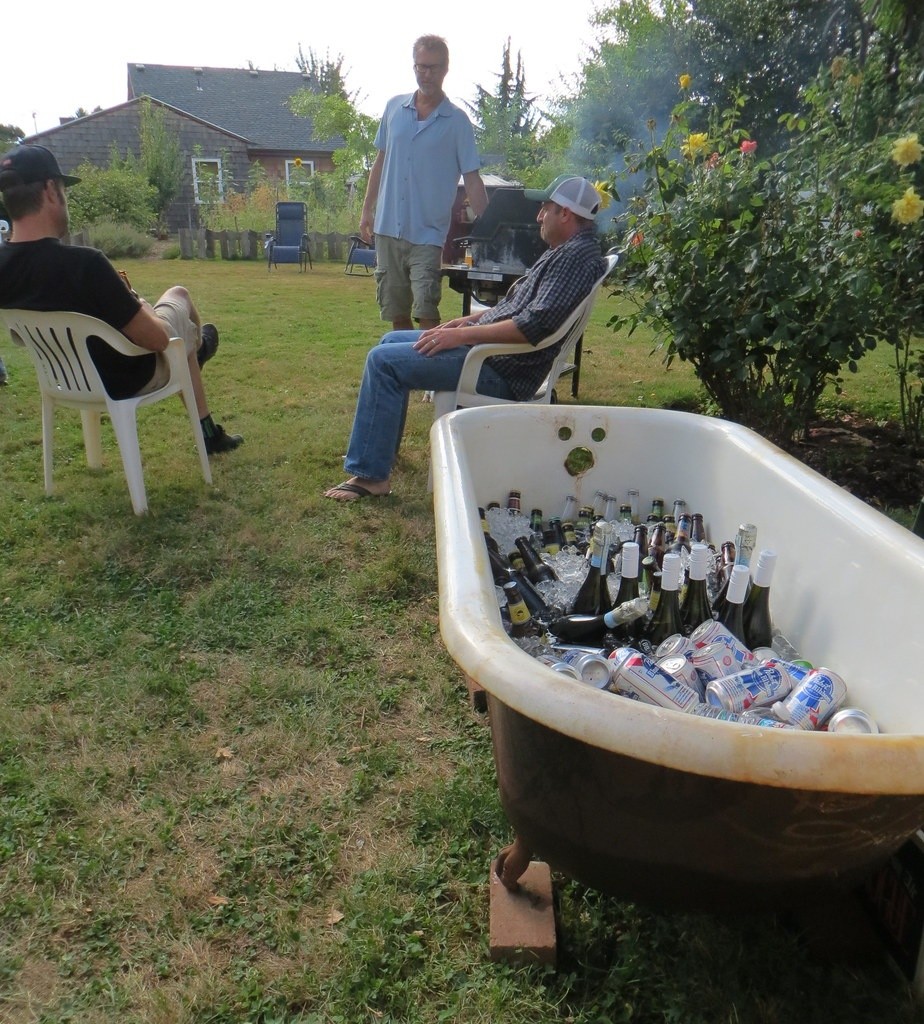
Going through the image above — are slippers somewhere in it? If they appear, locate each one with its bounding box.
[323,480,391,502]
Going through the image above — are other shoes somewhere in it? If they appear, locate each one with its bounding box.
[204,424,244,455]
[197,323,219,371]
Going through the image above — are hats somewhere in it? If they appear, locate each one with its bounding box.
[524,173,602,221]
[0,144,82,193]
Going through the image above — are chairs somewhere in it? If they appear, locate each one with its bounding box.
[343,235,378,277]
[426,254,620,493]
[0,308,214,517]
[264,201,313,272]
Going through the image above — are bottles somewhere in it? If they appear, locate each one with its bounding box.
[710,523,757,646]
[672,499,686,528]
[691,701,798,730]
[771,635,814,671]
[663,515,675,545]
[485,516,580,657]
[692,513,706,544]
[547,520,714,660]
[465,246,472,268]
[478,507,498,553]
[592,490,607,512]
[604,495,617,522]
[628,490,639,525]
[487,502,500,511]
[507,491,543,532]
[652,497,664,521]
[620,504,632,525]
[575,504,605,560]
[562,495,578,523]
[119,270,138,300]
[743,549,777,653]
[646,513,659,528]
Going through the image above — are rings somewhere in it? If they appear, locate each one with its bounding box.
[431,340,437,345]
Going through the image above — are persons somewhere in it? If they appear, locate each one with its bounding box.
[322,174,608,503]
[359,35,490,331]
[0,144,245,456]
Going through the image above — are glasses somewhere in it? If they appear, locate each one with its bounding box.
[414,63,446,73]
